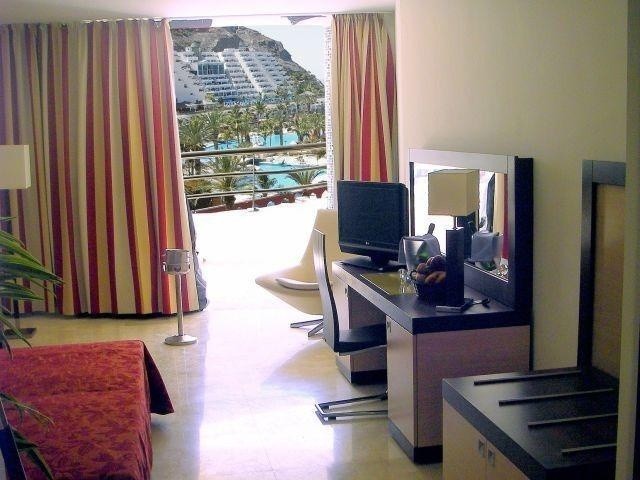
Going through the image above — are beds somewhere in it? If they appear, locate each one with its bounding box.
[1,339,176,479]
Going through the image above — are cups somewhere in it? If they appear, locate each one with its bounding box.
[471,231,504,270]
[403,239,430,273]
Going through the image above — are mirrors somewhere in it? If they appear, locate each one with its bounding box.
[403,146,533,309]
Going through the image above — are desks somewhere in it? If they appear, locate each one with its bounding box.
[328,258,535,464]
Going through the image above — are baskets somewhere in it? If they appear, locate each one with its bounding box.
[409,269,446,305]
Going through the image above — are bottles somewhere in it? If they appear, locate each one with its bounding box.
[414,223,436,266]
[468,215,496,270]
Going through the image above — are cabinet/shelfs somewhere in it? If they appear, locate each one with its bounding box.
[438,365,640,479]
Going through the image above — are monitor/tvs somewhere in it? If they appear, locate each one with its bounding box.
[337,180,409,273]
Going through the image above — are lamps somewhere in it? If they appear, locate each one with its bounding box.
[0,144,38,339]
[427,168,479,312]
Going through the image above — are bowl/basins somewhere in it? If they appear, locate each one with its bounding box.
[407,276,445,301]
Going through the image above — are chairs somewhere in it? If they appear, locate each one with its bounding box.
[258,209,339,336]
[307,225,387,420]
